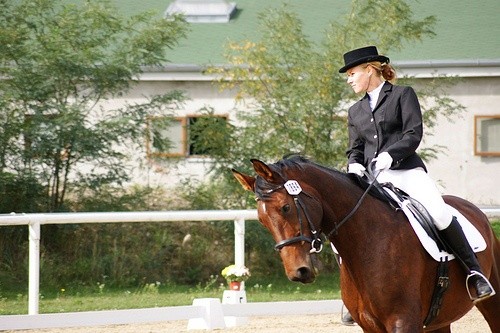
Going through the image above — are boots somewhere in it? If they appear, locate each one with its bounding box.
[439,216,492,298]
[342,310,353,322]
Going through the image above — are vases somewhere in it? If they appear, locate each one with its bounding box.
[228,283,240,290]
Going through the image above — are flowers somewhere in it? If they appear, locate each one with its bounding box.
[222,265,251,284]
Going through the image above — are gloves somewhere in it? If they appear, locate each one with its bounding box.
[347,162,366,177]
[375,151,393,171]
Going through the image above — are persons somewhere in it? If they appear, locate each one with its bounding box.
[339,46,492,322]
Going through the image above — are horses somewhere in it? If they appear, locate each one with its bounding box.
[229,151,499,332]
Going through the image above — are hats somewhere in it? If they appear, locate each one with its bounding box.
[339,46,389,73]
